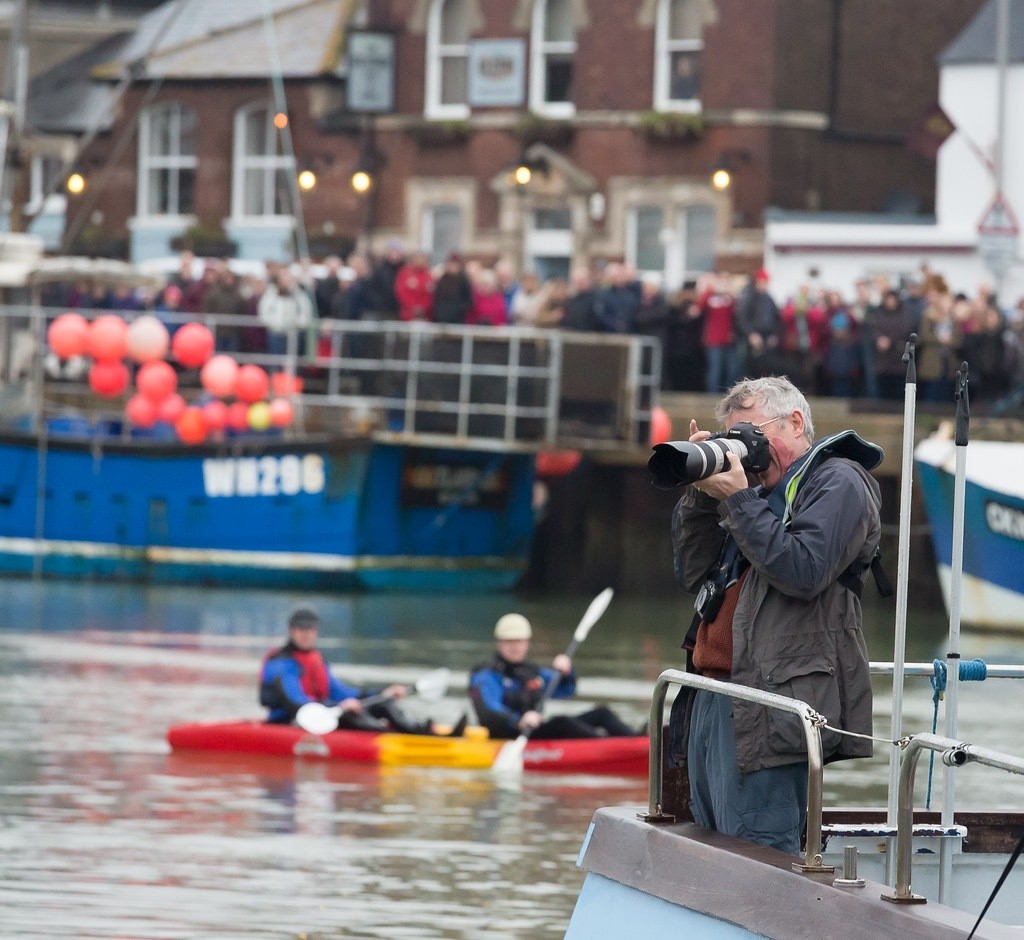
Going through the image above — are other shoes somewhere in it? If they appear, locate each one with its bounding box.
[451,715,467,737]
[634,721,650,736]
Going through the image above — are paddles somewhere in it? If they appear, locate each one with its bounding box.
[293,689,398,736]
[488,585,616,776]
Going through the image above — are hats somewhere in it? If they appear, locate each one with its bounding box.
[752,268,768,280]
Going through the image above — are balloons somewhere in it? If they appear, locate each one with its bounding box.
[51,311,300,447]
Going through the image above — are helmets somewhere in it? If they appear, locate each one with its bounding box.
[494,612,533,639]
[287,604,320,630]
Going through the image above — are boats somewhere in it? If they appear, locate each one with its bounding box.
[559,653,1024,938]
[158,712,650,782]
[0,299,665,589]
[913,416,1024,629]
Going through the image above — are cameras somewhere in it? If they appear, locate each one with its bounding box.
[694,579,725,623]
[648,422,772,492]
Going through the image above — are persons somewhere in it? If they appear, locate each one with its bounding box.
[596,260,1024,417]
[469,614,649,740]
[260,610,468,737]
[33,250,638,391]
[667,375,884,860]
